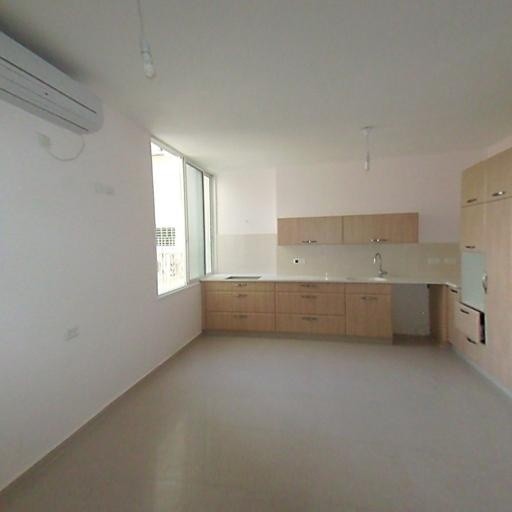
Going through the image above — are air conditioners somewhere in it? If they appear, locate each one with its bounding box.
[0,28,106,135]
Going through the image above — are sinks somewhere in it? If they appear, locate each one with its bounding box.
[344,276,387,282]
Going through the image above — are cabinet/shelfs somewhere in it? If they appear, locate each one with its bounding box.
[341,212,419,244]
[346,285,394,337]
[274,283,346,333]
[446,285,460,349]
[277,217,342,244]
[482,147,511,395]
[203,281,275,334]
[459,161,482,254]
[452,300,484,371]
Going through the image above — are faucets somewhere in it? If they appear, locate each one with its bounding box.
[373,253,381,272]
